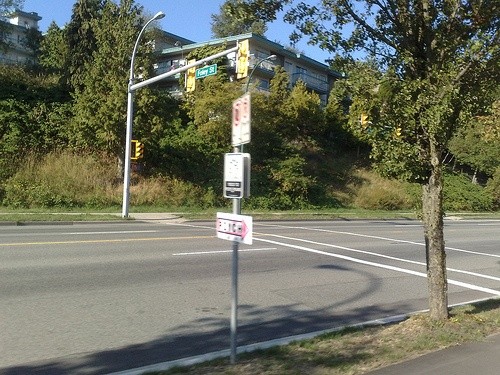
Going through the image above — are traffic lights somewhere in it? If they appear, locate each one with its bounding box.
[360,112,369,130]
[235,38,250,80]
[187,59,196,93]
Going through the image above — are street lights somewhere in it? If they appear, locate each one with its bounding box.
[240,54,278,154]
[122,11,165,220]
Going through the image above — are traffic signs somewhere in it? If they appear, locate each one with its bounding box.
[216,92,253,246]
